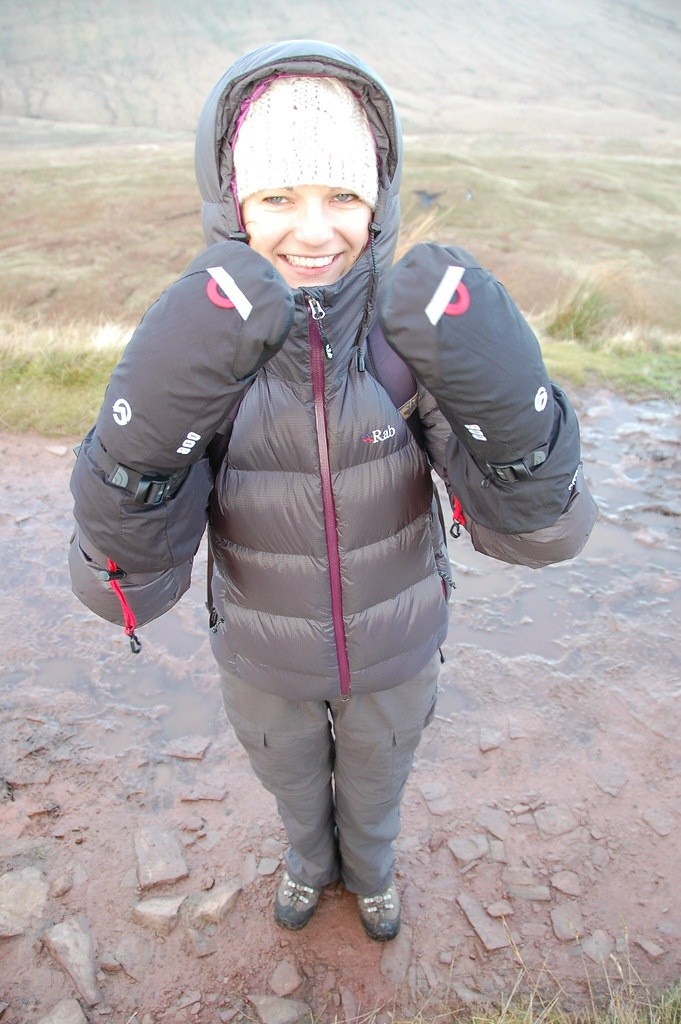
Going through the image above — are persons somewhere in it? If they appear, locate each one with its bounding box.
[68,39,597,944]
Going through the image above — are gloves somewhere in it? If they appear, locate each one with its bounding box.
[67,240,294,571]
[377,241,579,536]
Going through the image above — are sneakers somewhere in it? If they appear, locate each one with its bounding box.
[274,872,322,931]
[355,881,400,941]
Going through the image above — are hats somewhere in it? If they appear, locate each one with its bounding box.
[232,76,378,211]
